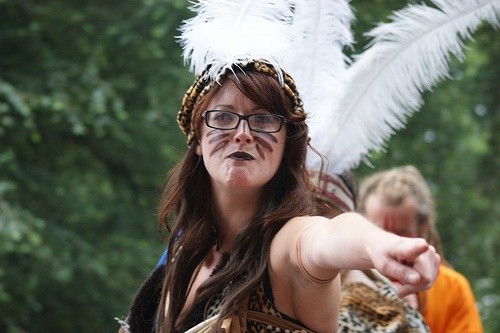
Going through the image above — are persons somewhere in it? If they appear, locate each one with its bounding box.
[308,169,431,333]
[357,166,484,333]
[113,57,440,333]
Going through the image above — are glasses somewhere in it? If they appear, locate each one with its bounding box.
[202,110,288,133]
